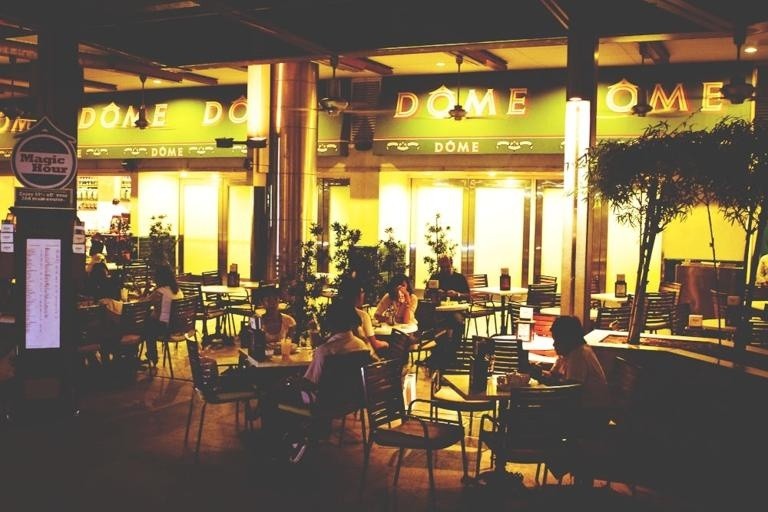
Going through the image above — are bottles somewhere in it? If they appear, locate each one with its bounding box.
[446,296,451,305]
[389,305,394,325]
[385,307,390,324]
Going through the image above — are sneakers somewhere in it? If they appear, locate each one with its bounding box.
[289,440,308,465]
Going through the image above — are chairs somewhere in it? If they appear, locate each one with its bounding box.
[463,274,498,336]
[527,283,557,304]
[378,328,410,359]
[357,356,469,497]
[202,271,223,285]
[671,303,690,334]
[535,274,556,283]
[311,350,371,460]
[473,384,581,487]
[415,300,449,382]
[585,355,643,495]
[664,259,683,281]
[507,300,541,335]
[155,295,201,378]
[121,264,149,297]
[423,369,473,436]
[711,289,727,318]
[184,332,263,466]
[118,300,154,374]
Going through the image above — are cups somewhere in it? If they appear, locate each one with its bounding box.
[281,338,291,361]
[121,288,128,302]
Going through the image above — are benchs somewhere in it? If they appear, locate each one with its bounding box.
[628,291,676,335]
[176,281,230,335]
[596,306,632,330]
[683,323,736,341]
[656,281,683,314]
[430,337,523,376]
[745,287,768,345]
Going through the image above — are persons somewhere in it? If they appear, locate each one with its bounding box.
[373,273,419,336]
[343,280,390,361]
[143,267,185,361]
[91,264,118,298]
[529,316,607,413]
[424,253,468,326]
[84,238,104,271]
[264,303,368,463]
[252,287,297,344]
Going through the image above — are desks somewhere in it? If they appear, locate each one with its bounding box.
[591,292,636,308]
[202,283,250,336]
[675,263,744,315]
[417,296,469,312]
[439,374,581,430]
[238,345,319,370]
[364,307,418,337]
[470,283,529,332]
[102,262,149,273]
[242,280,265,288]
